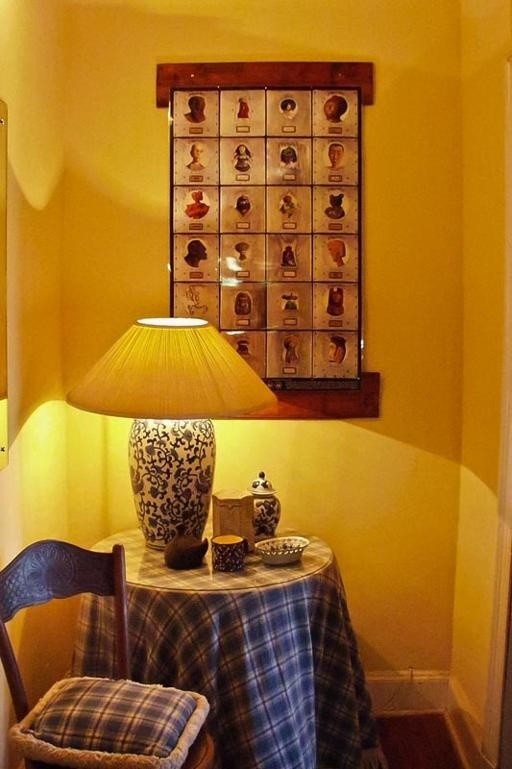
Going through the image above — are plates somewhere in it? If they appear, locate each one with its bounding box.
[254,537,309,566]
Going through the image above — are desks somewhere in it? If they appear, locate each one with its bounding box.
[72,524,391,768]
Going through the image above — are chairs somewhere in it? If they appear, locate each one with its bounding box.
[0,538,217,769]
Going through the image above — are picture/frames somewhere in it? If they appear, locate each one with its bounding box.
[166,84,365,395]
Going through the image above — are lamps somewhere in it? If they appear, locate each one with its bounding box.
[64,314,279,553]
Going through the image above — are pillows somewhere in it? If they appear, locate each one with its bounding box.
[8,674,211,769]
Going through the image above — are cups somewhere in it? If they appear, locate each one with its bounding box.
[211,534,249,572]
